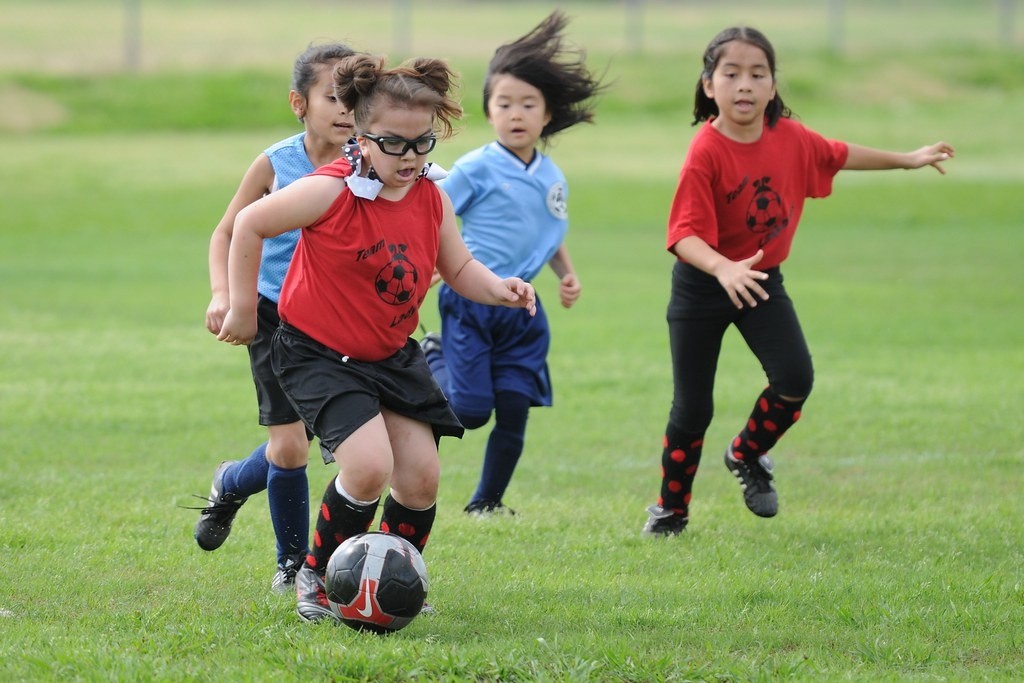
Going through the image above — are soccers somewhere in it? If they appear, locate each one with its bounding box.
[323,530,432,638]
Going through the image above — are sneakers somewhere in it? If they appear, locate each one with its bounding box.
[722,441,780,518]
[195,459,248,550]
[293,564,333,627]
[643,505,688,540]
[466,497,517,516]
[273,554,307,592]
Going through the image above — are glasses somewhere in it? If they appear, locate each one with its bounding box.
[364,132,439,157]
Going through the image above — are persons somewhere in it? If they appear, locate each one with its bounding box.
[215,52,536,628]
[177,38,356,595]
[643,26,953,533]
[418,8,620,520]
